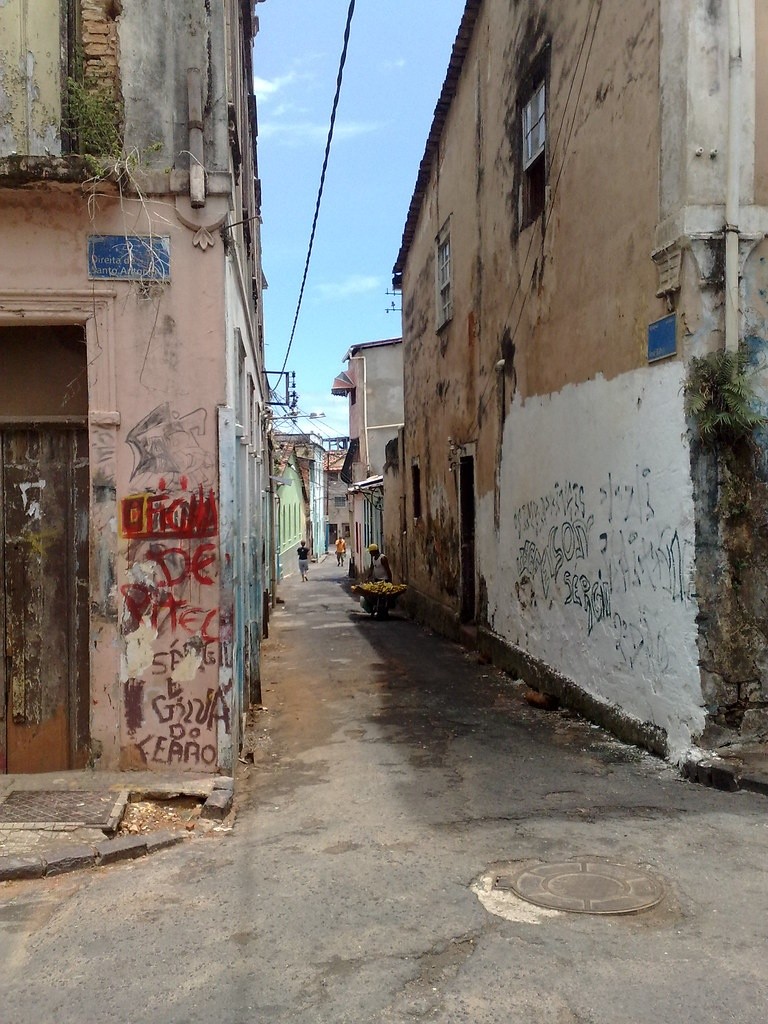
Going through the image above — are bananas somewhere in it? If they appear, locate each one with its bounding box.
[362,581,407,594]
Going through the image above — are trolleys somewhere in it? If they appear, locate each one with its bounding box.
[367,592,400,620]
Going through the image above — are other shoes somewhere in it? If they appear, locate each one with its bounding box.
[338,562,340,566]
[301,580,304,582]
[304,576,308,581]
[341,563,343,566]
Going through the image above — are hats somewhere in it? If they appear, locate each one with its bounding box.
[339,536,343,539]
[365,543,379,552]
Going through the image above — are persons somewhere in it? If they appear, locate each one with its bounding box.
[366,544,393,585]
[335,536,346,567]
[297,540,310,582]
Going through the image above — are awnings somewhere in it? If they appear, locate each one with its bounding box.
[331,369,356,396]
